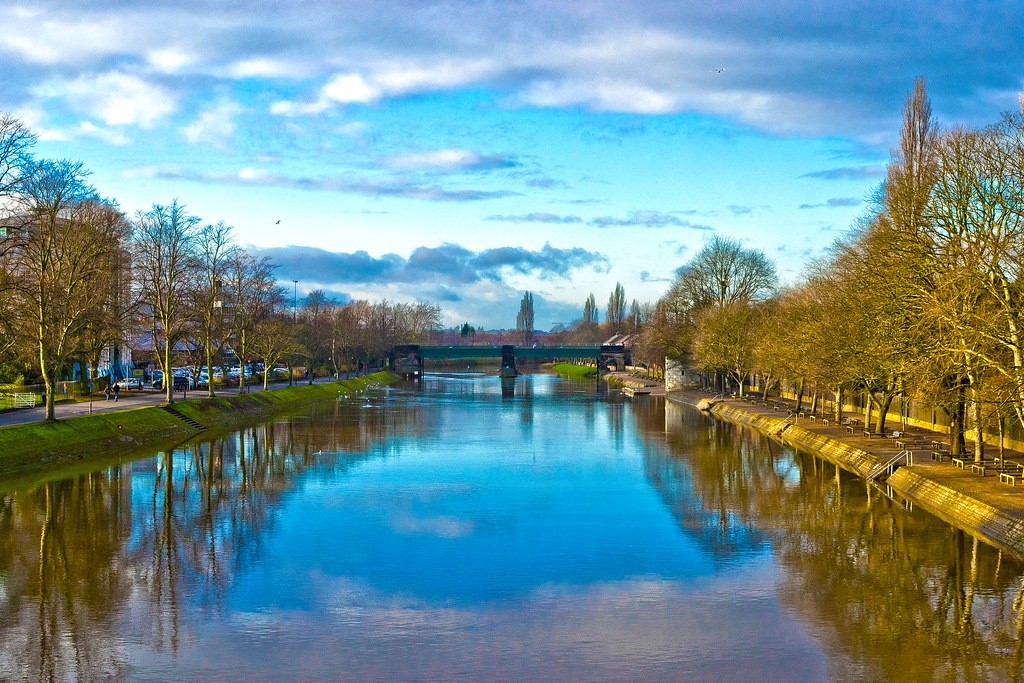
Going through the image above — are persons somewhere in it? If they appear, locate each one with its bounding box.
[333,371,337,381]
[104,382,121,402]
[41,389,48,407]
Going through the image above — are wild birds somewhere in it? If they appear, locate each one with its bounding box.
[274,219,283,227]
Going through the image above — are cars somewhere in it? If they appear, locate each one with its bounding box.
[148,363,288,385]
[152,376,189,390]
[113,378,144,391]
